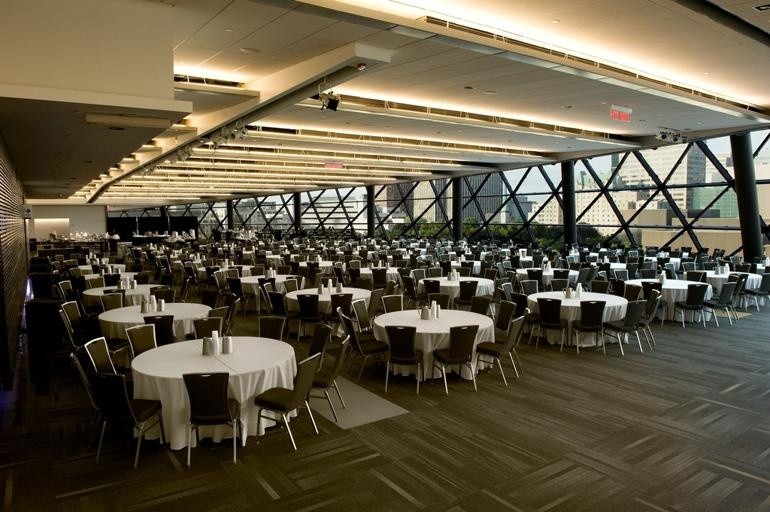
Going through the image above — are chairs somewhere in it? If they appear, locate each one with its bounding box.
[183,372,242,466]
[87,371,165,467]
[66,349,102,450]
[494,300,525,377]
[304,321,333,403]
[384,323,425,394]
[337,307,388,383]
[313,335,352,422]
[83,336,117,374]
[432,325,477,393]
[43,227,770,354]
[476,316,526,384]
[252,352,323,451]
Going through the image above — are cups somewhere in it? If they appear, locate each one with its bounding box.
[211,331,220,355]
[222,336,233,353]
[437,305,441,318]
[431,300,437,319]
[345,232,730,280]
[202,336,213,356]
[575,283,583,299]
[79,227,344,314]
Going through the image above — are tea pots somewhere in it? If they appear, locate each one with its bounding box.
[417,304,430,319]
[563,287,574,298]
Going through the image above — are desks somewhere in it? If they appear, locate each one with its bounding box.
[373,308,495,380]
[130,333,298,446]
